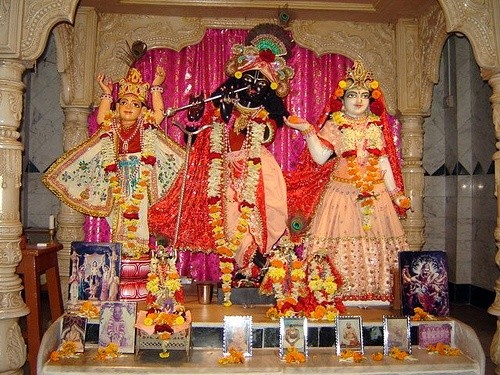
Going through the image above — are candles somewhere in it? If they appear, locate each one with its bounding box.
[49,215,54,229]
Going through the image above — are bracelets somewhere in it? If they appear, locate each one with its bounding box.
[151,85,163,93]
[101,93,111,99]
[388,187,403,200]
[301,125,316,139]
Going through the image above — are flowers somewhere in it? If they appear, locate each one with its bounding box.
[425,342,461,356]
[47,340,78,362]
[389,347,417,361]
[338,350,368,363]
[334,80,381,100]
[144,258,185,358]
[92,343,120,362]
[412,307,438,321]
[266,244,346,322]
[280,345,307,366]
[217,349,245,367]
[234,70,242,79]
[371,351,382,361]
[73,298,101,319]
[270,82,278,90]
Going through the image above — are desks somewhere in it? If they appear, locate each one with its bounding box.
[14,242,64,375]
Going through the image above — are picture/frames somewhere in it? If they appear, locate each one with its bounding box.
[334,315,364,356]
[223,316,253,357]
[382,314,412,356]
[279,316,308,358]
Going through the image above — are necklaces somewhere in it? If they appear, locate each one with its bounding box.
[222,107,264,189]
[113,119,144,182]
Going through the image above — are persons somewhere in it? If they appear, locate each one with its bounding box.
[421,327,449,343]
[150,23,294,282]
[340,322,360,347]
[284,324,304,348]
[229,326,246,351]
[43,64,186,256]
[69,254,119,300]
[100,305,129,347]
[283,59,414,301]
[62,325,82,349]
